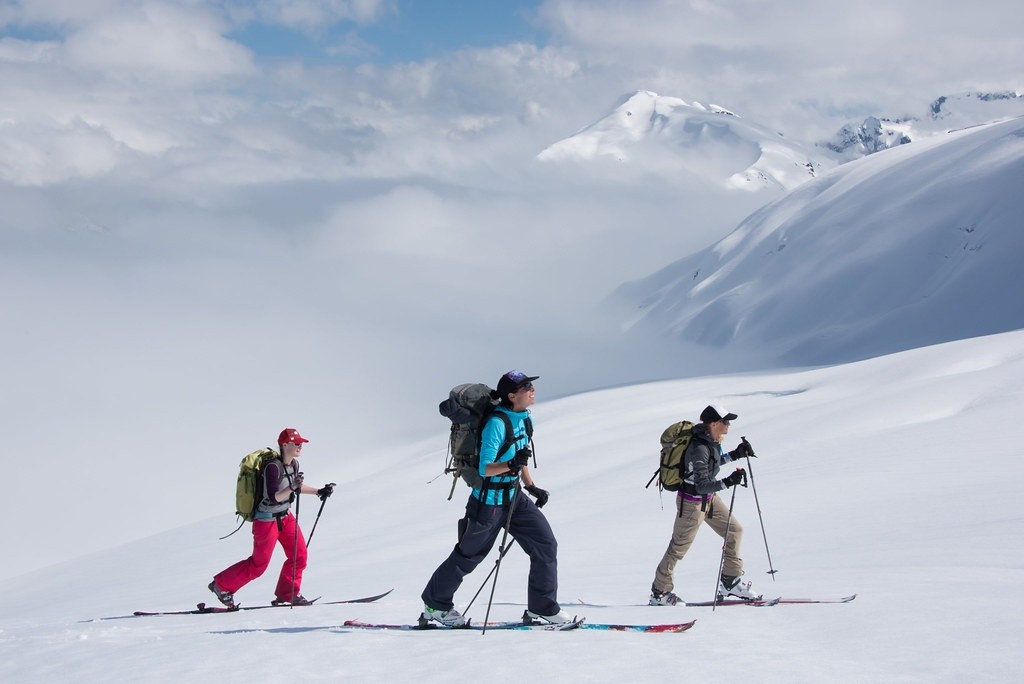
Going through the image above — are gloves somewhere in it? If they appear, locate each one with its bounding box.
[523,481,549,508]
[318,484,334,501]
[722,471,745,489]
[507,448,532,474]
[729,441,754,461]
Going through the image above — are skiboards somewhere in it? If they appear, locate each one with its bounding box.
[577,593,858,607]
[340,617,699,634]
[78,586,396,624]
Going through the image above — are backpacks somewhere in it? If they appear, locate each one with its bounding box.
[438,383,533,490]
[236,447,296,522]
[659,421,714,492]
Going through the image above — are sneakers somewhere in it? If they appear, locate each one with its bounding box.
[522,608,574,625]
[423,602,466,627]
[719,576,768,602]
[649,592,686,607]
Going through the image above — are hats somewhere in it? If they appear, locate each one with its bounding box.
[497,370,539,398]
[700,404,737,422]
[277,428,309,445]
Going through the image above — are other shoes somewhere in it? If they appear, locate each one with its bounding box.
[276,593,308,604]
[208,579,234,607]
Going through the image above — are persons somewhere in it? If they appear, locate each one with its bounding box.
[421,369,572,628]
[207,428,333,607]
[649,404,757,606]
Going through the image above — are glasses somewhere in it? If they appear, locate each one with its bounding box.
[288,442,302,447]
[714,419,730,425]
[513,383,533,393]
